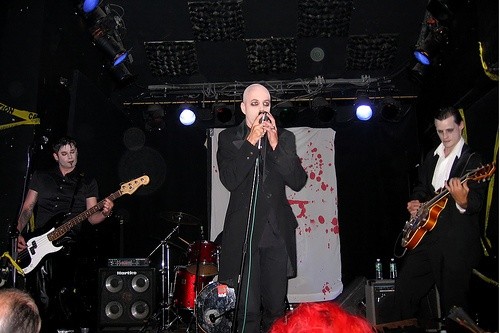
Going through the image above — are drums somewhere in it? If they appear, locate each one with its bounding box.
[185,240,219,277]
[194,281,239,333]
[170,264,211,313]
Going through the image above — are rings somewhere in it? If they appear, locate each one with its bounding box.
[271,126,275,130]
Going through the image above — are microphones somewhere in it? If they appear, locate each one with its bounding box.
[257,111,269,150]
[209,314,216,323]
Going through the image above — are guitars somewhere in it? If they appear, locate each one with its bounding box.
[401,160,496,251]
[16,174,150,277]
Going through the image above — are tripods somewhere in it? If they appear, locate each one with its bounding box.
[136,221,193,333]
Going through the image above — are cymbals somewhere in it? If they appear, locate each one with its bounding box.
[157,210,200,226]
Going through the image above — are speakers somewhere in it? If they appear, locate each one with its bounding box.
[364,279,441,333]
[98,267,162,326]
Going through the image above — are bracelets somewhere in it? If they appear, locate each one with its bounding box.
[102,211,113,218]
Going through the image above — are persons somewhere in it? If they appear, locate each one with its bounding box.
[16,136,114,305]
[216,84,309,333]
[0,288,42,333]
[398,105,493,333]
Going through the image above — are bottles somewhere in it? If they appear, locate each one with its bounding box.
[389,259,397,279]
[376,259,384,280]
